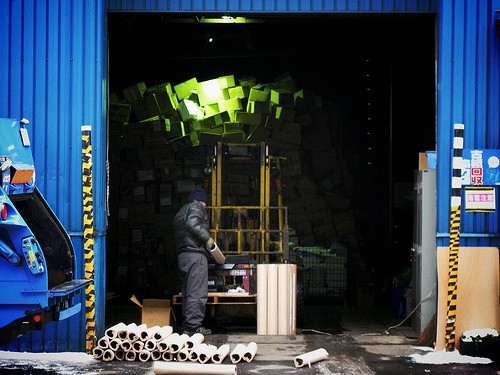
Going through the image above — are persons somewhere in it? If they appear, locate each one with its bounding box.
[172,185,214,335]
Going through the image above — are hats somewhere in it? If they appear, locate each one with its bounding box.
[190,186,208,202]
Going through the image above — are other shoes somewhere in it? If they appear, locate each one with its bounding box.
[183,327,211,335]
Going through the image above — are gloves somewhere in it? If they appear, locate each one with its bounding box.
[206,237,214,251]
